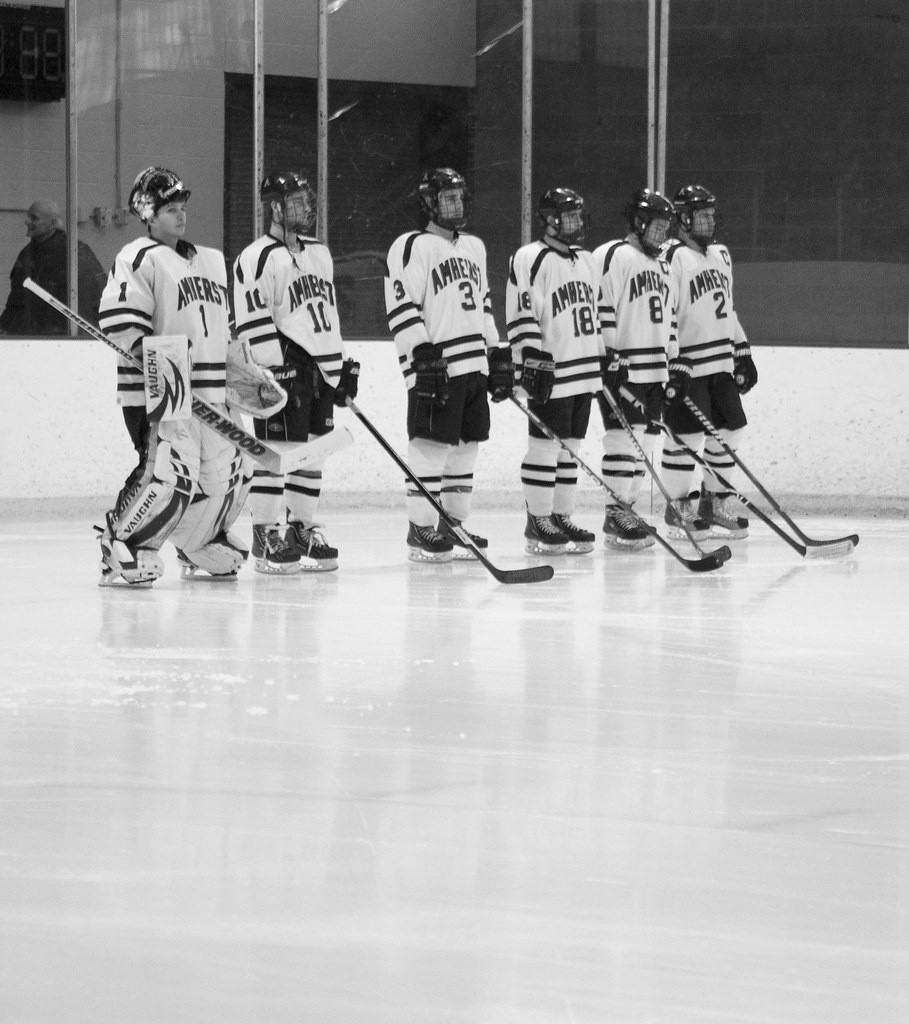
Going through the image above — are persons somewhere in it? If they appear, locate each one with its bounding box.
[0,199,107,335]
[98,165,288,590]
[383,168,514,565]
[505,187,623,554]
[656,184,758,540]
[589,190,692,551]
[233,171,361,574]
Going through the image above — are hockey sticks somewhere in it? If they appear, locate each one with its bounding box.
[344,397,556,586]
[682,395,861,548]
[508,394,723,573]
[602,384,733,564]
[22,275,356,478]
[619,386,856,560]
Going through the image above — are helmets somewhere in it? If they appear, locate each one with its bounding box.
[539,187,584,223]
[674,184,716,218]
[625,187,677,236]
[418,167,465,200]
[128,166,191,230]
[260,171,307,211]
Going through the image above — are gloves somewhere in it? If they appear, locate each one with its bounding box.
[592,348,619,398]
[332,360,361,407]
[734,342,758,395]
[613,352,630,401]
[487,346,515,402]
[665,357,694,406]
[272,363,300,415]
[520,348,556,405]
[411,344,449,408]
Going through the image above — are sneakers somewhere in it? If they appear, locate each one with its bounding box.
[665,490,710,543]
[523,499,570,556]
[284,506,339,571]
[437,499,489,560]
[406,520,456,563]
[602,501,656,552]
[251,522,301,575]
[549,512,596,554]
[175,545,238,582]
[697,481,749,539]
[98,537,159,589]
